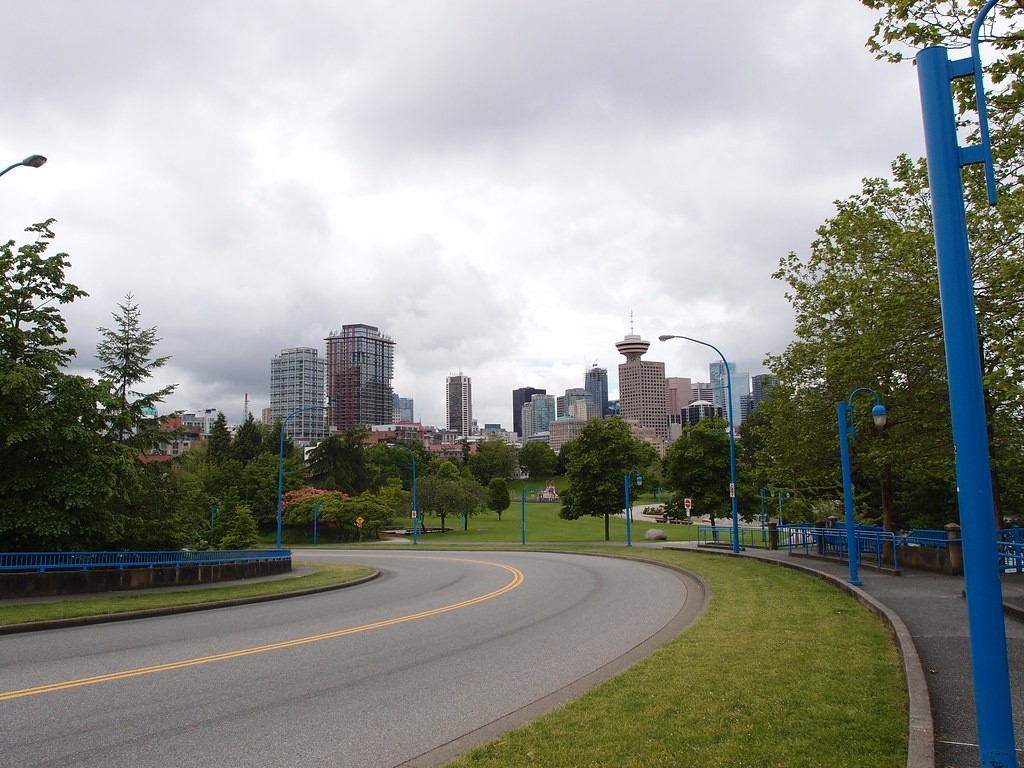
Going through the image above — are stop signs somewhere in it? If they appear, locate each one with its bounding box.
[684,498,691,509]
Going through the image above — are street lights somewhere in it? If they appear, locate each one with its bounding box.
[387,443,416,543]
[658,335,738,554]
[312,502,324,546]
[521,485,535,544]
[276,405,331,547]
[837,385,888,586]
[212,505,220,531]
[779,491,791,531]
[624,470,641,546]
[761,488,775,542]
